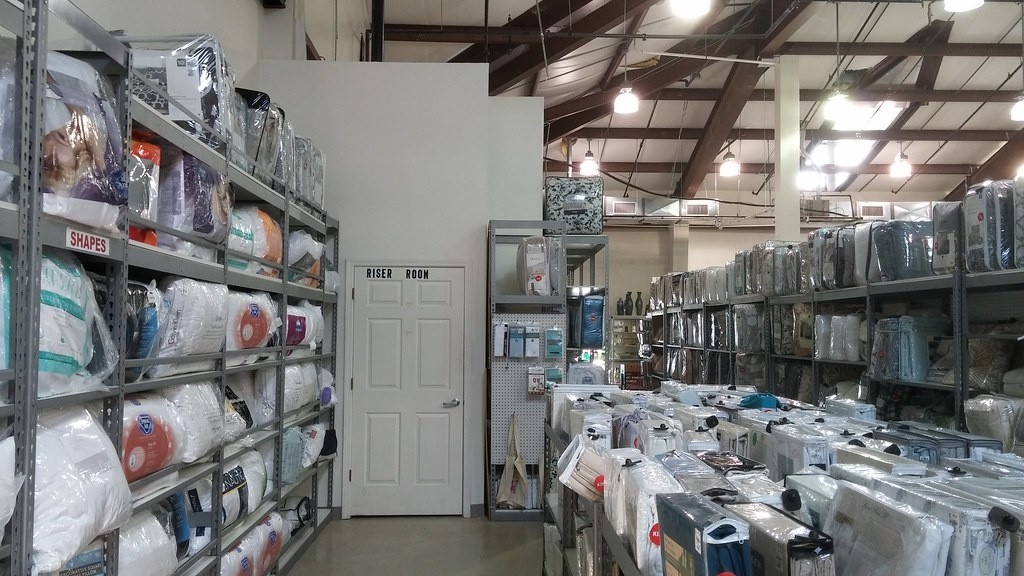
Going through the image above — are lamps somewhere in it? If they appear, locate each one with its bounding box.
[719,139,740,176]
[580,138,599,176]
[824,0,858,120]
[614,49,638,113]
[889,141,911,178]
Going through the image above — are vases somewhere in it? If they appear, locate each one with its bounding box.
[635,292,643,316]
[617,292,633,316]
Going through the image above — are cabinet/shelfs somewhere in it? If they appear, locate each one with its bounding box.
[0,1,340,576]
[545,234,1024,576]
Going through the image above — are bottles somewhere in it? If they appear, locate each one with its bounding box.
[624,292,633,315]
[617,298,623,315]
[635,292,643,315]
